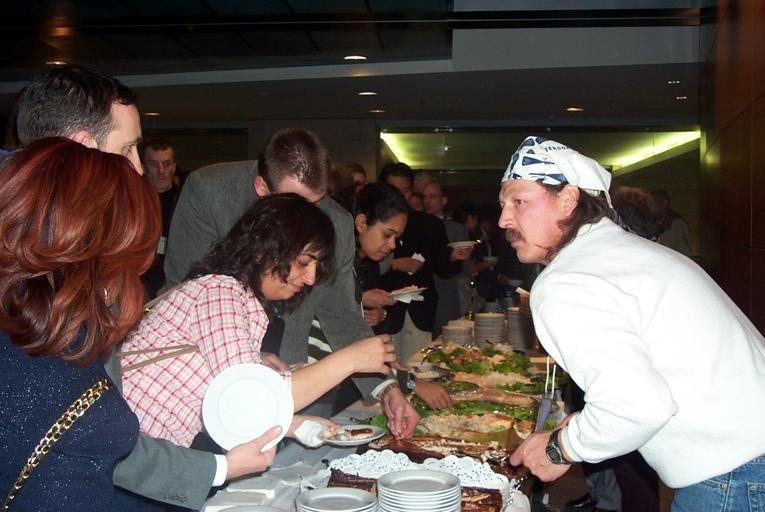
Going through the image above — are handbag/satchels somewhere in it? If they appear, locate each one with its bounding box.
[100,350,123,395]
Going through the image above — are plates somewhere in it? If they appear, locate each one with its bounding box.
[482,256,500,261]
[474,312,505,348]
[446,240,476,250]
[506,307,526,352]
[442,325,472,346]
[201,363,294,452]
[376,469,462,511]
[416,376,441,382]
[388,286,427,298]
[294,486,376,511]
[317,424,385,446]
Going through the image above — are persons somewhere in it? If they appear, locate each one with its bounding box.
[0,83,488,511]
[498,135,765,512]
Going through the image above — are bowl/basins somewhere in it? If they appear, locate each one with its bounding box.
[509,280,524,287]
[406,361,433,371]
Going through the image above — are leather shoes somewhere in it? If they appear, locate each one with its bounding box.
[566,494,599,510]
